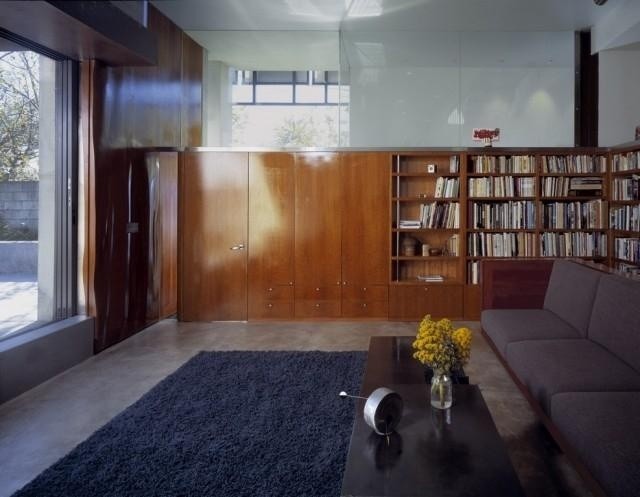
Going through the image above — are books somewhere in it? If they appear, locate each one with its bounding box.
[399,149,640,285]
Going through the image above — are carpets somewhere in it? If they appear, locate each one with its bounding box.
[12,350,367,497]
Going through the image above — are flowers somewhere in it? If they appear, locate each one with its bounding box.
[413,315,472,407]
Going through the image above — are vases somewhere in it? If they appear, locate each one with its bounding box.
[431,370,453,409]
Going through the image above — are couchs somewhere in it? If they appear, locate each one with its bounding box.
[478,258,640,496]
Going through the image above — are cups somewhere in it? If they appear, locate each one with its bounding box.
[422,244,430,257]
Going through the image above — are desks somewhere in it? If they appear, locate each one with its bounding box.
[342,336,522,495]
